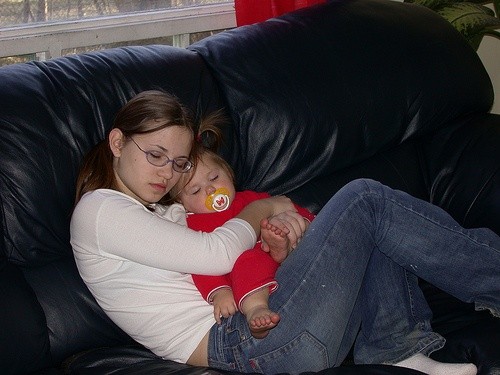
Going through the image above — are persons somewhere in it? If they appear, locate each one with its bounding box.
[168,111,316,339]
[69,88,500,375]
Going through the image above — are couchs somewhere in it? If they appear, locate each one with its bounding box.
[0,0,500,375]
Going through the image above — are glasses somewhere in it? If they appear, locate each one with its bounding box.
[125,134,193,173]
